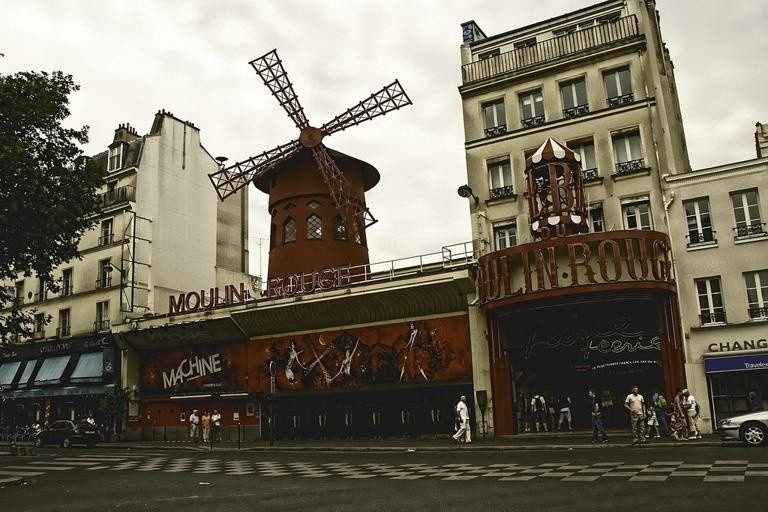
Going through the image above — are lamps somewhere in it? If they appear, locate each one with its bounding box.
[103,262,126,279]
[458,184,479,207]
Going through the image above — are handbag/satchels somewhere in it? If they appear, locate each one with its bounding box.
[673,405,686,418]
[692,404,700,419]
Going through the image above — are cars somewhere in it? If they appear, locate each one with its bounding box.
[34,419,100,449]
[714,410,768,445]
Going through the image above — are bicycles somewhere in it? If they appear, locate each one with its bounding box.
[6,422,36,443]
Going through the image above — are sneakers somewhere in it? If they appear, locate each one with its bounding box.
[654,435,660,438]
[688,436,697,440]
[544,430,549,432]
[602,439,608,443]
[697,434,702,439]
[525,428,531,432]
[632,438,639,443]
[536,430,541,433]
[671,433,687,441]
[640,438,647,444]
[644,434,650,438]
[591,440,598,444]
[452,435,475,444]
[190,438,222,443]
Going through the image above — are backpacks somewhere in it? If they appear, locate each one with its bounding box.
[558,399,569,408]
[655,394,668,411]
[532,395,543,413]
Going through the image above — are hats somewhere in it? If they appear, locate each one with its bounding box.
[193,409,199,414]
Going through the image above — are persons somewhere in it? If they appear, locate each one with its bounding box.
[518,385,701,444]
[746,382,764,411]
[452,395,473,444]
[189,409,222,444]
[31,422,39,435]
[87,416,95,426]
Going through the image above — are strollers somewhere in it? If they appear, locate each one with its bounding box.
[664,410,686,442]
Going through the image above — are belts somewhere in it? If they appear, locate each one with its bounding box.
[192,423,199,425]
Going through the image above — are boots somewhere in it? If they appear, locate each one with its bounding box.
[557,423,562,432]
[568,422,573,431]
